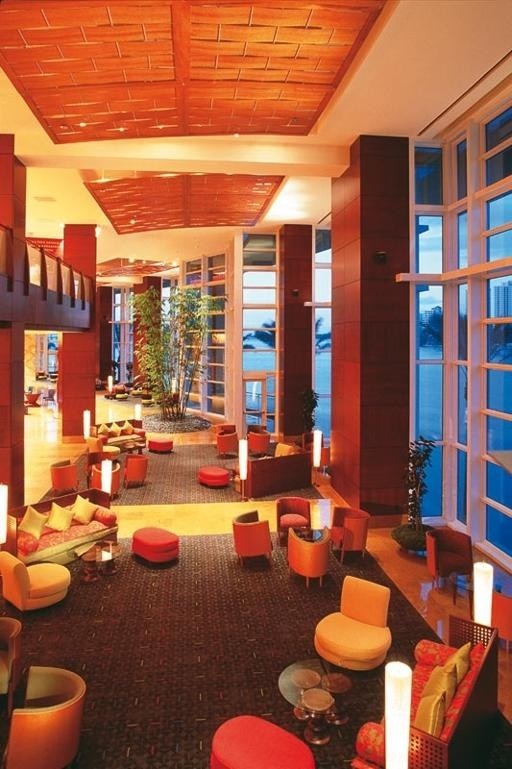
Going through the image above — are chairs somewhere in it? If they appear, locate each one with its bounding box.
[36,369,147,405]
[330,506,369,564]
[314,574,391,671]
[0,550,70,611]
[273,496,312,547]
[0,616,22,715]
[491,591,512,652]
[287,526,332,588]
[196,425,331,501]
[2,489,119,566]
[50,423,174,498]
[4,666,85,768]
[426,529,473,590]
[232,510,275,567]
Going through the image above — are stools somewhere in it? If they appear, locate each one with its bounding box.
[132,527,180,562]
[210,715,315,768]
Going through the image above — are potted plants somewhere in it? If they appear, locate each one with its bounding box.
[391,435,437,552]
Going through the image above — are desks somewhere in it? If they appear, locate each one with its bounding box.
[67,540,130,582]
[278,657,367,747]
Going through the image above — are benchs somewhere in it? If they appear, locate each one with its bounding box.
[351,614,499,769]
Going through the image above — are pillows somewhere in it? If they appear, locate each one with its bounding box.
[16,505,48,540]
[414,638,472,738]
[71,494,99,526]
[44,501,75,532]
